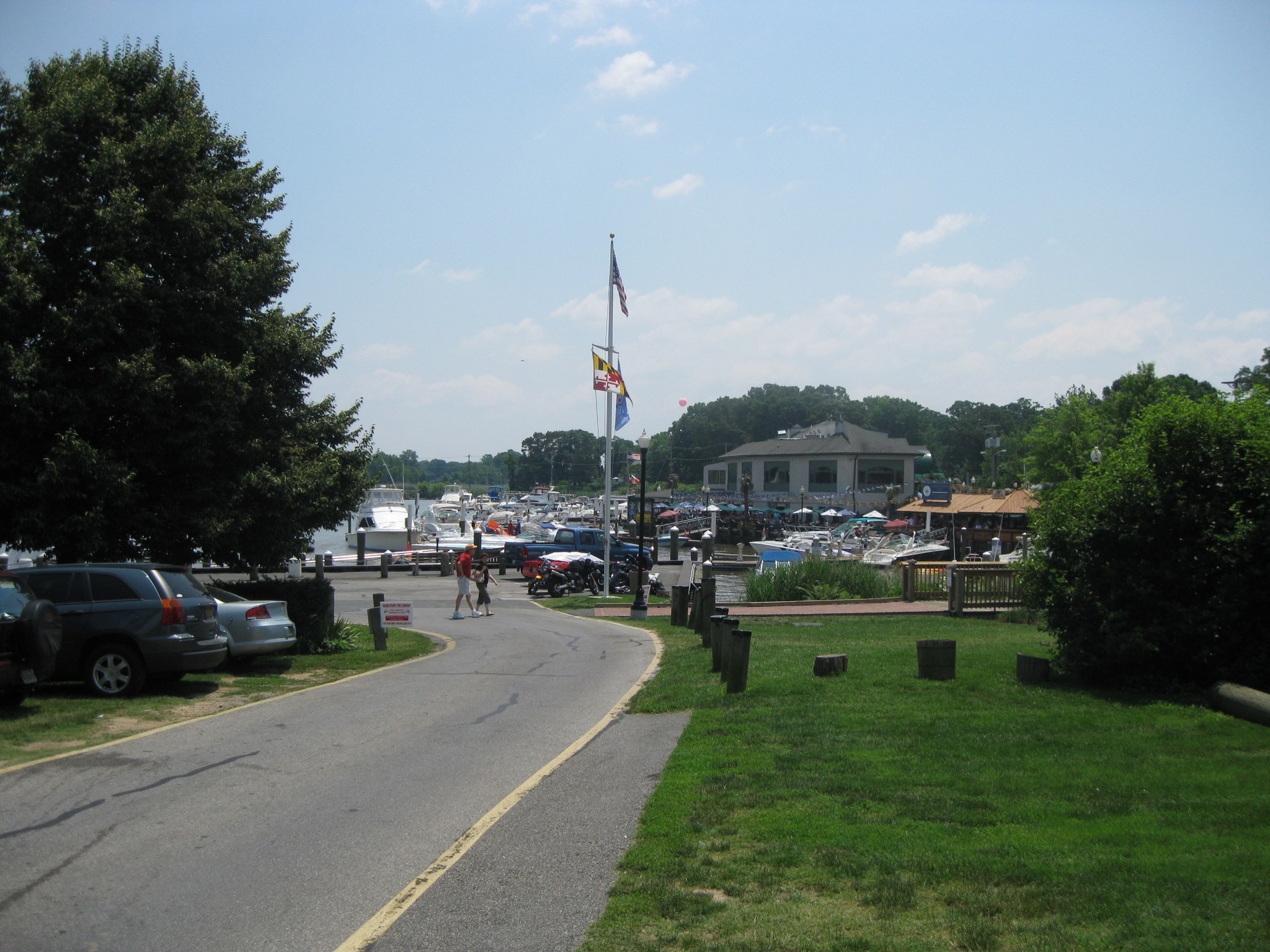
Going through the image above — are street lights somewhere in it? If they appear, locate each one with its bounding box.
[800,485,806,525]
[701,485,711,526]
[628,429,651,620]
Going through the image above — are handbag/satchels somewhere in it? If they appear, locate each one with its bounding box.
[470,564,485,584]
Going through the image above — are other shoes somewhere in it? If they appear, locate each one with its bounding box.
[485,612,495,616]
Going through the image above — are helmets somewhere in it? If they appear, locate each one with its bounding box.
[585,559,594,568]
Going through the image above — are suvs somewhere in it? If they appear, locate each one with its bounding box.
[0,572,66,705]
[0,562,227,696]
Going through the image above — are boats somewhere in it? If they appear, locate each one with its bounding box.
[411,483,628,550]
[345,488,422,551]
[749,522,955,563]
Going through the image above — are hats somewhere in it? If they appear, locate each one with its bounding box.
[464,544,479,550]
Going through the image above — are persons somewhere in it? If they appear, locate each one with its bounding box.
[507,519,521,536]
[452,543,498,619]
[683,509,795,530]
[420,514,427,523]
[471,517,489,534]
[895,513,1029,531]
[817,514,850,529]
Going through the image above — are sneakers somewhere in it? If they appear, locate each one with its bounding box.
[453,612,464,619]
[472,610,482,618]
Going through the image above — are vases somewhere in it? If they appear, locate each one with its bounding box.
[916,639,956,680]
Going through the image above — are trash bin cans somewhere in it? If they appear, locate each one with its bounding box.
[671,585,689,626]
[289,558,302,578]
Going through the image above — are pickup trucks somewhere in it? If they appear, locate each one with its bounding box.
[504,527,654,571]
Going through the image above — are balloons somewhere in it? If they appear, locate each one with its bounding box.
[679,398,687,407]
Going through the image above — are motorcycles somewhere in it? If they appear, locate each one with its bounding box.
[528,552,600,598]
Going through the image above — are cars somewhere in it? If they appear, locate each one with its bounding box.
[522,551,604,577]
[205,584,297,656]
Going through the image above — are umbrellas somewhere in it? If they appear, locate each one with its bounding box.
[883,518,907,533]
[655,501,887,521]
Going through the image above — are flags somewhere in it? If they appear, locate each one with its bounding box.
[615,359,630,431]
[627,452,641,461]
[628,473,640,485]
[613,248,629,317]
[593,352,634,407]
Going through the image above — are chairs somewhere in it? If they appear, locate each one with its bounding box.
[586,535,593,545]
